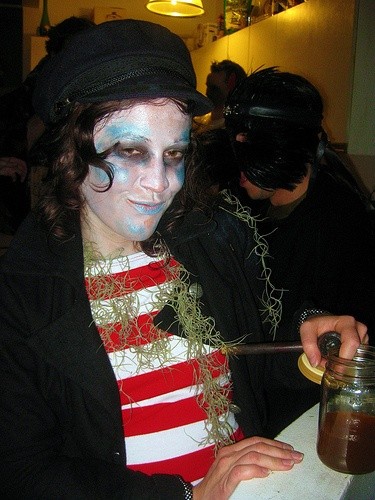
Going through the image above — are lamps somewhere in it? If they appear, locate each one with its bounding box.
[146,0,205,18]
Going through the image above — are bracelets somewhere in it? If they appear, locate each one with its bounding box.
[178,477,193,500]
[297,308,332,336]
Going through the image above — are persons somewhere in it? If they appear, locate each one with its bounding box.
[193,60,248,135]
[0,157,27,183]
[0,20,369,500]
[13,16,96,222]
[186,72,375,353]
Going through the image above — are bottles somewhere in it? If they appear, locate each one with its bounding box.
[38,0,50,36]
[316,343,375,475]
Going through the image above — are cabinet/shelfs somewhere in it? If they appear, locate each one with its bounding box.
[189,0,375,155]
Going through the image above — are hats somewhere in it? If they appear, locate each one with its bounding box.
[24,15,214,120]
